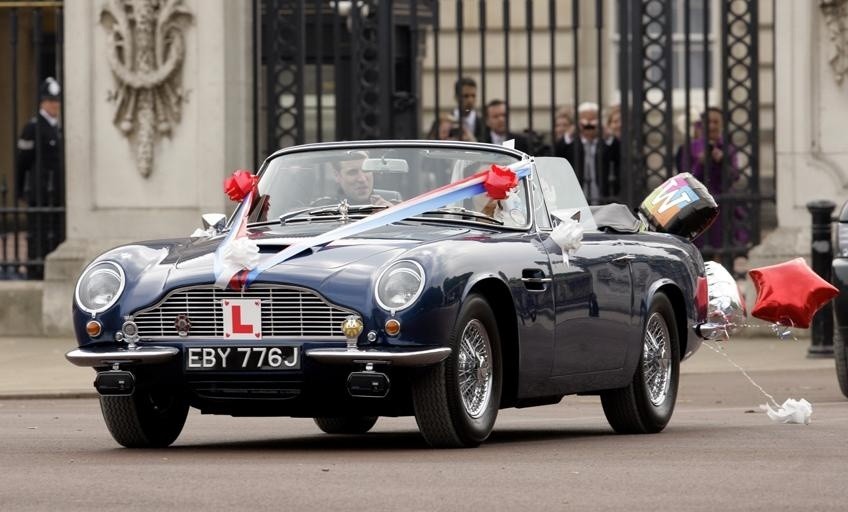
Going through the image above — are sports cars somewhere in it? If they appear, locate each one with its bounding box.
[62,136,714,449]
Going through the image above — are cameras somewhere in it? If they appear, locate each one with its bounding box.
[583,124,596,129]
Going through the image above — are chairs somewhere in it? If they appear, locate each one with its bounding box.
[373,189,401,206]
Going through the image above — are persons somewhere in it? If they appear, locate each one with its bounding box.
[329,150,403,213]
[13,74,65,278]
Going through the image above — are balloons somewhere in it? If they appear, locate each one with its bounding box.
[703,251,750,347]
[748,255,845,332]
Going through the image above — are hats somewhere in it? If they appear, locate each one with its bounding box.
[39,75,63,104]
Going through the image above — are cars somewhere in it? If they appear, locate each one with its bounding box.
[828,194,848,395]
